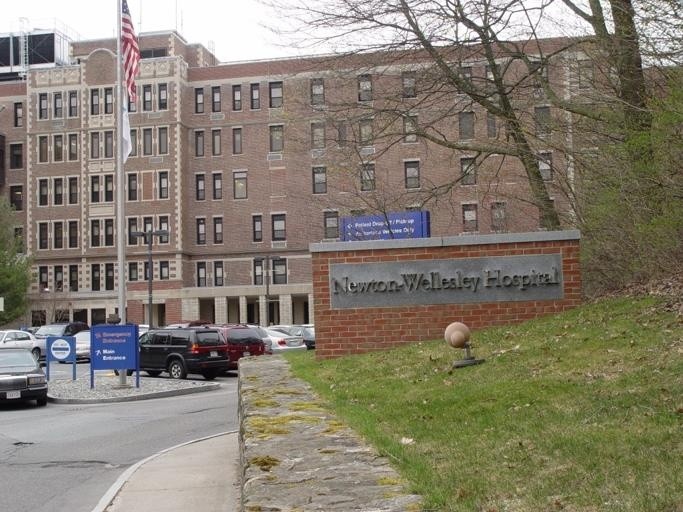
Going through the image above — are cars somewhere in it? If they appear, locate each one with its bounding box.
[0,346,52,409]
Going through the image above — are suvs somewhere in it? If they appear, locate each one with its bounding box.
[112,324,230,380]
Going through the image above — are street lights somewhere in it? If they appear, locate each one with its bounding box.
[128,228,169,329]
[252,254,280,325]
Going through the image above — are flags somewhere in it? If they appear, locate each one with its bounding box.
[122,92,132,162]
[120,0,140,103]
